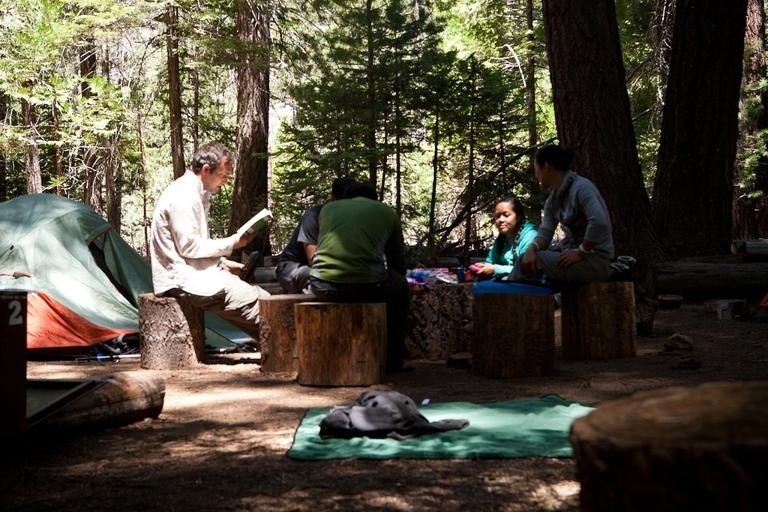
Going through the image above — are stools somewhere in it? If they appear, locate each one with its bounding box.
[560,281,638,361]
[258,294,317,374]
[294,302,387,387]
[471,281,555,379]
[138,292,204,370]
[569,379,768,512]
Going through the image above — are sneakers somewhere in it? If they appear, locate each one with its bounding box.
[385,360,415,374]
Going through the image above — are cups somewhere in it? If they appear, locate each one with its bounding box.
[451,268,464,283]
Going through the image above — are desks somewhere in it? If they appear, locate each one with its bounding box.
[400,280,474,365]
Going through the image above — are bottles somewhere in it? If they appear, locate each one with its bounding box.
[239,251,259,280]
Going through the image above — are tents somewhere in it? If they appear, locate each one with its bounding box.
[1,190,259,357]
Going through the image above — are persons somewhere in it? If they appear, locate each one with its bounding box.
[465,190,539,280]
[274,175,361,294]
[148,142,276,341]
[308,180,412,378]
[502,143,616,290]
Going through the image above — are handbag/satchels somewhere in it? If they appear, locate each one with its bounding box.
[664,330,704,368]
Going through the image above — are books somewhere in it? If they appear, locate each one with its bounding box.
[235,208,275,244]
